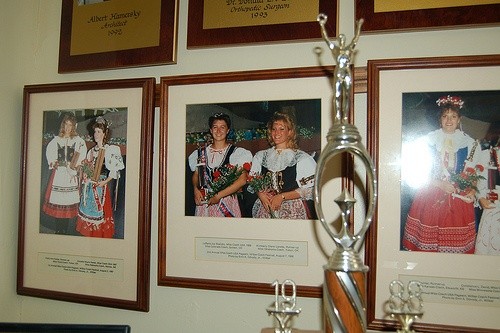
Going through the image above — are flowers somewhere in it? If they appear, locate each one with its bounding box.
[49,158,68,170]
[429,163,486,210]
[76,159,94,183]
[203,162,251,206]
[246,171,277,201]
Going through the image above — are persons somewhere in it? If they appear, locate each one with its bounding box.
[188,113,254,218]
[42,112,87,235]
[474,147,500,256]
[402,95,482,254]
[76,116,125,238]
[248,112,316,219]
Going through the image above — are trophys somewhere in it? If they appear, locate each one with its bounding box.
[381,280,424,333]
[314,13,378,333]
[265,279,302,333]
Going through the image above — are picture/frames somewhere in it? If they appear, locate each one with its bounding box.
[354,0,500,37]
[362,53,500,333]
[156,65,368,299]
[185,0,340,50]
[57,0,179,74]
[17,76,156,314]
